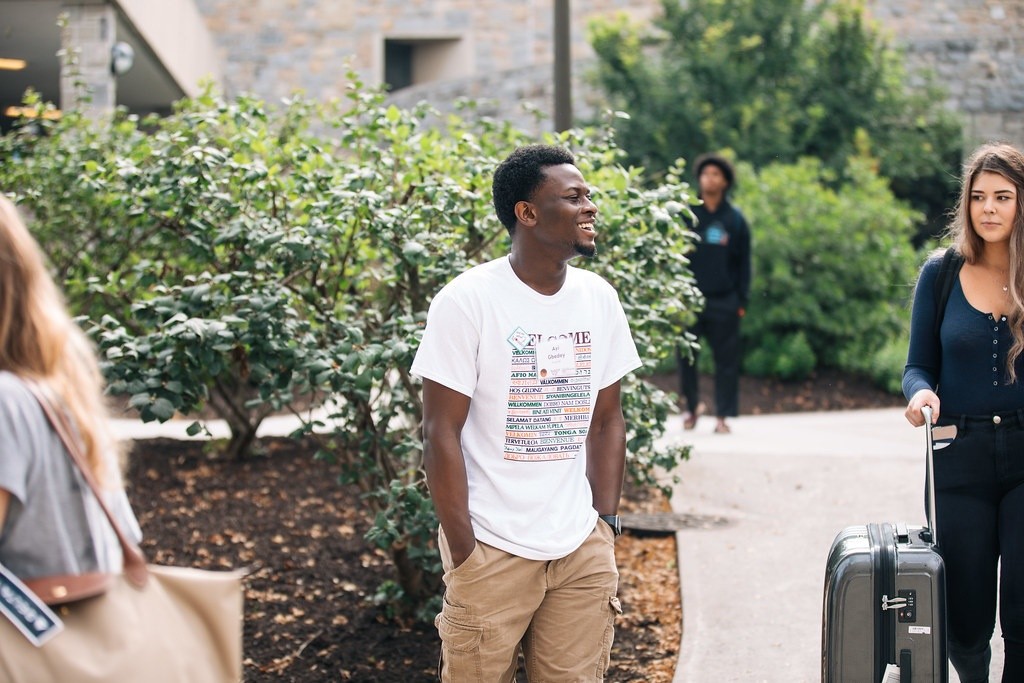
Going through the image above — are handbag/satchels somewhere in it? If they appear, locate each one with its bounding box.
[0,382,246,683]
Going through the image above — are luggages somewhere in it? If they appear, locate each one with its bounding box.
[820,407,948,683]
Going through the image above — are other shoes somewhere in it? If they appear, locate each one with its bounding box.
[683,413,696,428]
[715,423,727,433]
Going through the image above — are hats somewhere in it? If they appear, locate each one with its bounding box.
[693,153,734,191]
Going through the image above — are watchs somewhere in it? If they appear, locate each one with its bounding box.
[601,515,622,535]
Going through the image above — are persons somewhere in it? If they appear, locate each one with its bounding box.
[0,192,145,583]
[406,143,643,683]
[899,141,1024,683]
[673,155,754,432]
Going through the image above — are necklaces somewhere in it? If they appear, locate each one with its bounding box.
[983,256,1010,291]
